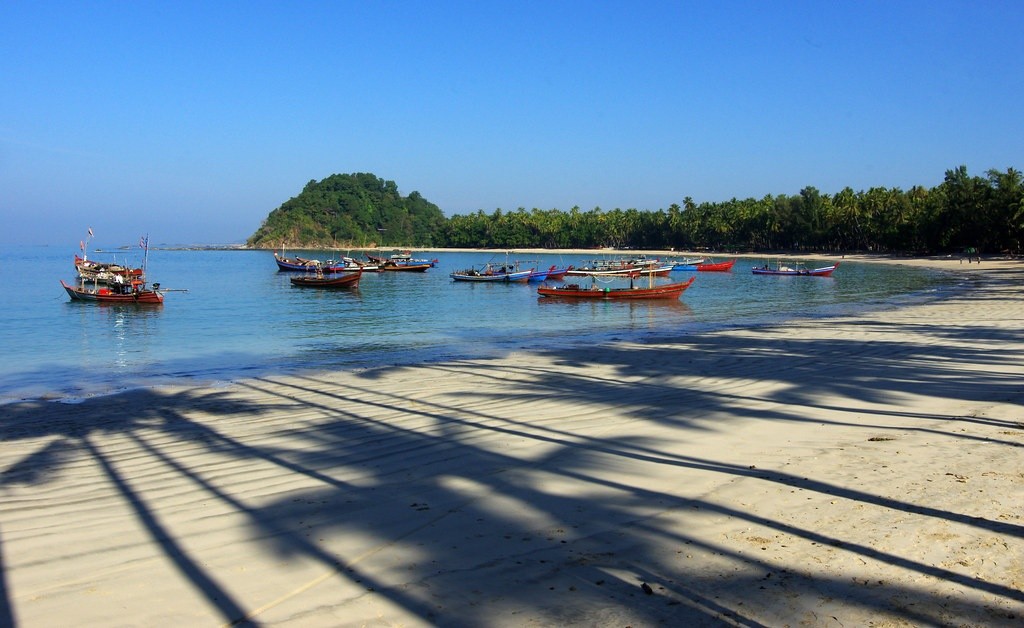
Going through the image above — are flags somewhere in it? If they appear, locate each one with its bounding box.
[140,237,144,248]
[80,242,84,250]
[88,228,92,236]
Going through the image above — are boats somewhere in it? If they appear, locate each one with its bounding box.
[751,257,841,277]
[450,252,573,283]
[273,243,439,288]
[537,275,696,299]
[60,227,165,303]
[567,253,736,276]
